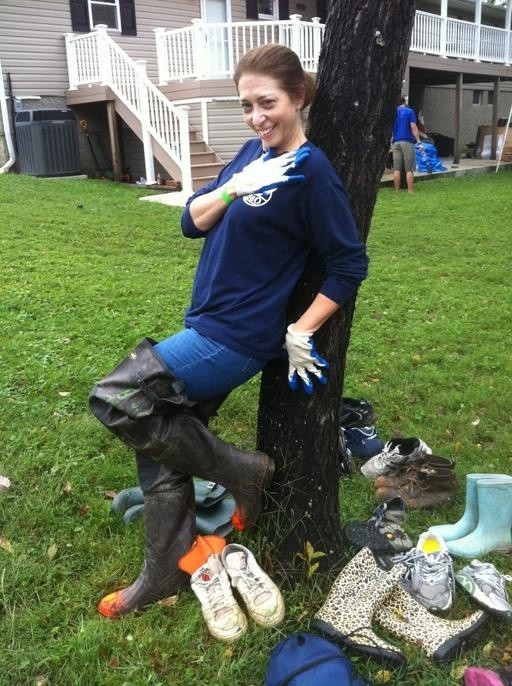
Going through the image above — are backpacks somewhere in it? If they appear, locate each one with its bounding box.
[262,630,367,686]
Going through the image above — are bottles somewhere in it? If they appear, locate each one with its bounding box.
[428,163,432,173]
[420,151,426,162]
[157,172,162,184]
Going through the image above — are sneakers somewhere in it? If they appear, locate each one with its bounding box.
[341,397,378,426]
[390,532,456,612]
[454,558,512,617]
[190,552,248,644]
[463,664,511,686]
[220,542,286,628]
[367,496,409,529]
[344,426,383,455]
[361,437,432,478]
[343,521,413,553]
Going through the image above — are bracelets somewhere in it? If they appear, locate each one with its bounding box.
[417,141,421,145]
[218,182,232,205]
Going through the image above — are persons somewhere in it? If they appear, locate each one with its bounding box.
[87,47,371,616]
[390,97,424,194]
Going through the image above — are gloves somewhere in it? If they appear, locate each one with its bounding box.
[282,325,331,394]
[231,146,312,197]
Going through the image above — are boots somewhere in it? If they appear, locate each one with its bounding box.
[88,337,276,531]
[375,464,458,509]
[123,499,236,539]
[371,583,491,661]
[98,445,196,618]
[446,478,512,558]
[312,547,407,662]
[112,481,227,515]
[428,473,508,542]
[374,451,455,489]
